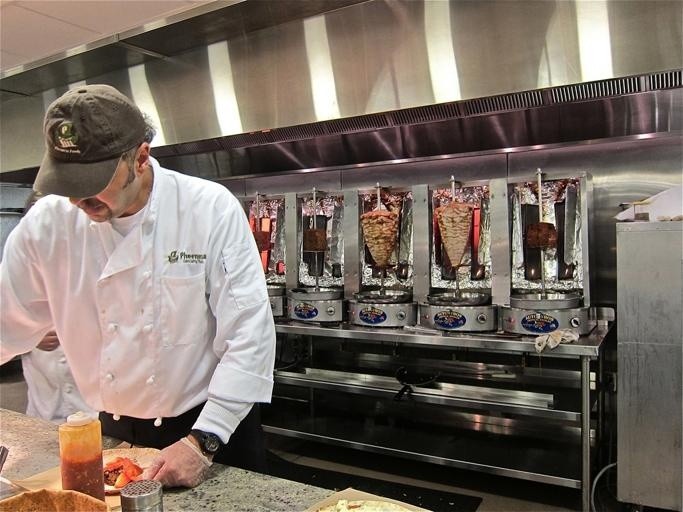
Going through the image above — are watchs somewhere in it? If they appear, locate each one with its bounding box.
[189,428,222,457]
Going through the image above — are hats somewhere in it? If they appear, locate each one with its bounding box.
[32,83,147,197]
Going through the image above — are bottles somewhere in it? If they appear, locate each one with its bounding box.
[121,478,164,512]
[56,411,107,509]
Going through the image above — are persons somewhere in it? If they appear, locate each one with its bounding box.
[19,190,96,426]
[1,84,277,493]
